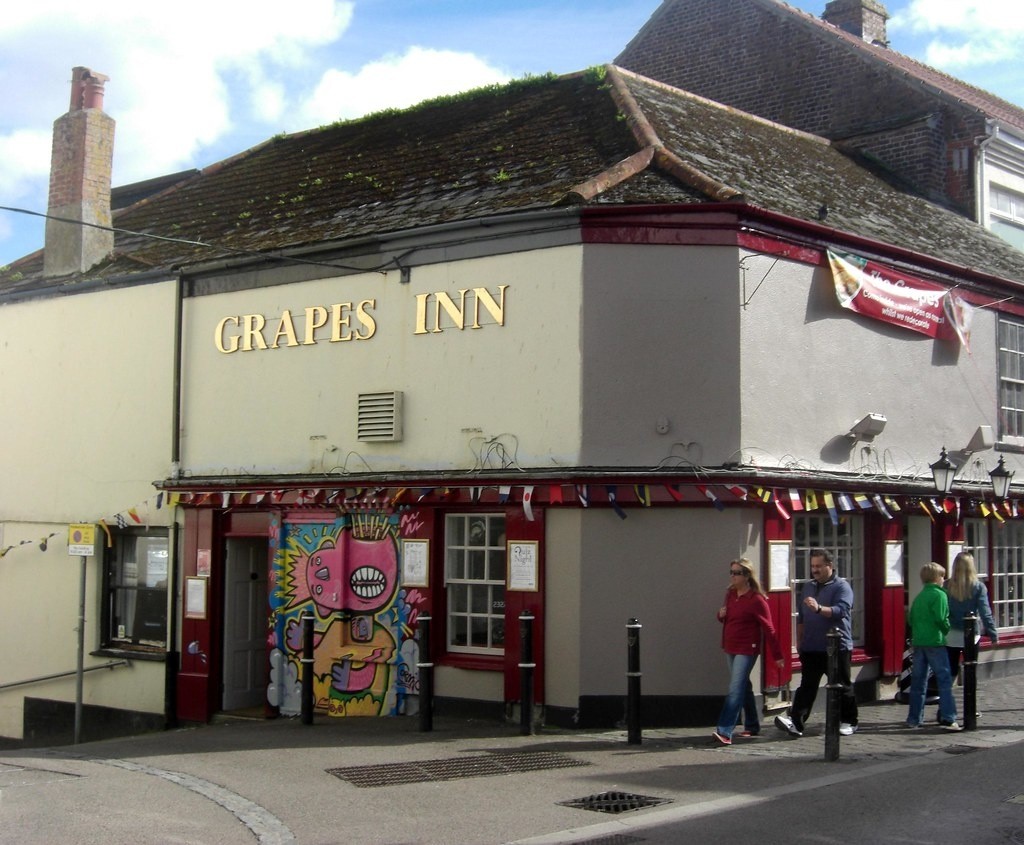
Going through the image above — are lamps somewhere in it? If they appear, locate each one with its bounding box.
[845,412,887,443]
[988,452,1015,504]
[929,443,958,499]
[960,425,994,456]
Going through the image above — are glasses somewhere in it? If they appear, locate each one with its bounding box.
[730,570,743,575]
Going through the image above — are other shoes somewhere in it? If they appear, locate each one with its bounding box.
[939,722,964,730]
[976,712,982,718]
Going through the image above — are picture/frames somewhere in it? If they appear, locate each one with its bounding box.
[946,541,965,579]
[184,575,207,620]
[884,539,905,587]
[506,539,539,592]
[767,539,793,593]
[401,538,430,588]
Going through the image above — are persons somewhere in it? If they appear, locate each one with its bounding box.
[772,547,860,738]
[904,562,967,731]
[935,552,999,731]
[709,557,784,746]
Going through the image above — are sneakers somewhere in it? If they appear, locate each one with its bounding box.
[775,716,802,736]
[908,725,918,728]
[739,731,758,736]
[712,732,732,745]
[839,723,858,735]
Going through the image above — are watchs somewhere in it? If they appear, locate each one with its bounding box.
[815,604,822,614]
